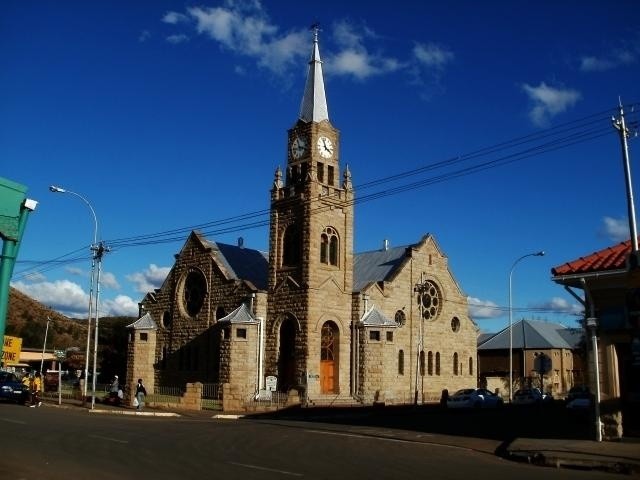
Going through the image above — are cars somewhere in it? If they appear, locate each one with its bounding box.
[567,393,595,412]
[0,371,29,405]
[511,388,543,408]
[446,387,503,412]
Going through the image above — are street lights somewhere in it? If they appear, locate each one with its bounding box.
[507,250,546,408]
[49,186,98,410]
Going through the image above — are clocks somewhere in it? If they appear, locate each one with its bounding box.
[291,137,305,159]
[317,137,334,159]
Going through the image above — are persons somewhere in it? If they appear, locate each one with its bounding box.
[21,372,31,403]
[109,375,119,406]
[31,371,42,407]
[134,379,144,411]
